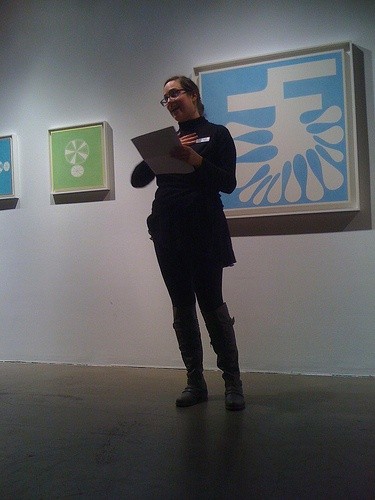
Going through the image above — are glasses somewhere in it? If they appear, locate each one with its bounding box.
[160,88,187,106]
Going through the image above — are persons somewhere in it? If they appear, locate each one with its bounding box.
[130,75,248,411]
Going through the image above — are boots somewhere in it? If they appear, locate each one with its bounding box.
[173,306,207,407]
[203,302,246,411]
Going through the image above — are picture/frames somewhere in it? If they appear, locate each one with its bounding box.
[1,132,20,204]
[48,120,112,196]
[185,40,366,220]
